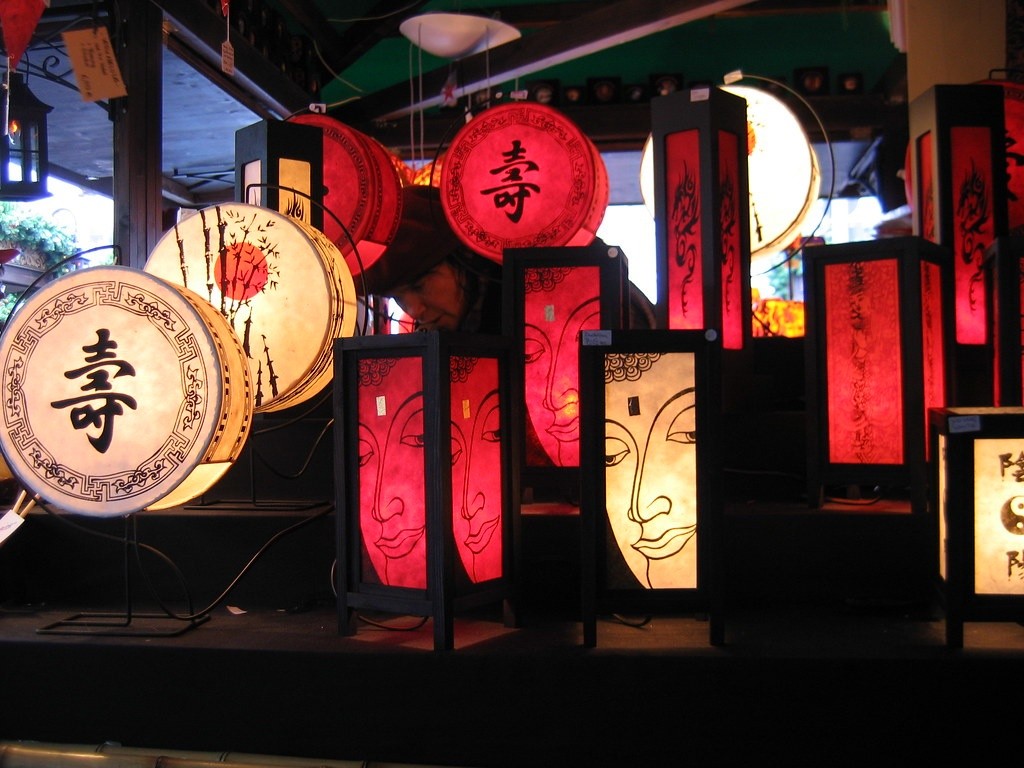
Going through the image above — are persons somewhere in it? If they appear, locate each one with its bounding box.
[358,185,659,371]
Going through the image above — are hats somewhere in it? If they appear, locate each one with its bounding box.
[354,185,464,297]
